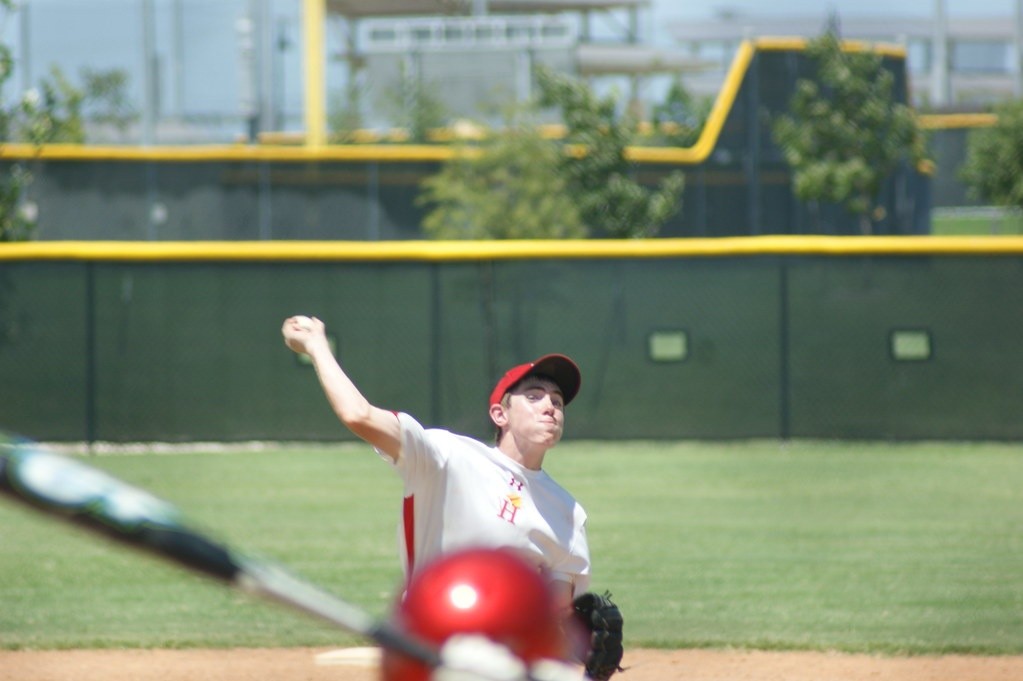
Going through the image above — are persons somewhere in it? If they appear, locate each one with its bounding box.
[282,314,591,680]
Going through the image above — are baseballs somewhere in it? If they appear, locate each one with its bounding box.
[293,315,313,329]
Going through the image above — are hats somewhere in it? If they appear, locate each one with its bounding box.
[489,353,584,411]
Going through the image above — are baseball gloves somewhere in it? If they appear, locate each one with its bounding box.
[571,592,624,681]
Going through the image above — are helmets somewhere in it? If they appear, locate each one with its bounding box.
[379,546,589,680]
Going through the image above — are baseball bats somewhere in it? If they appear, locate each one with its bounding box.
[0,429,443,665]
